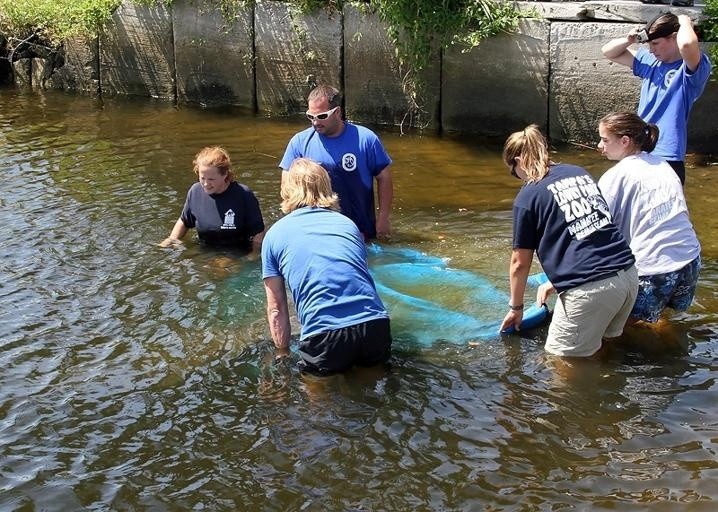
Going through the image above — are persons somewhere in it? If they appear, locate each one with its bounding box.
[602,11,712,194]
[279,84,395,244]
[590,110,702,332]
[496,123,640,377]
[157,145,266,278]
[261,155,391,395]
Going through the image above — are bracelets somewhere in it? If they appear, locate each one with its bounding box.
[509,303,524,310]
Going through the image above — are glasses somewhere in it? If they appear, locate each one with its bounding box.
[305,107,339,121]
[511,162,520,178]
